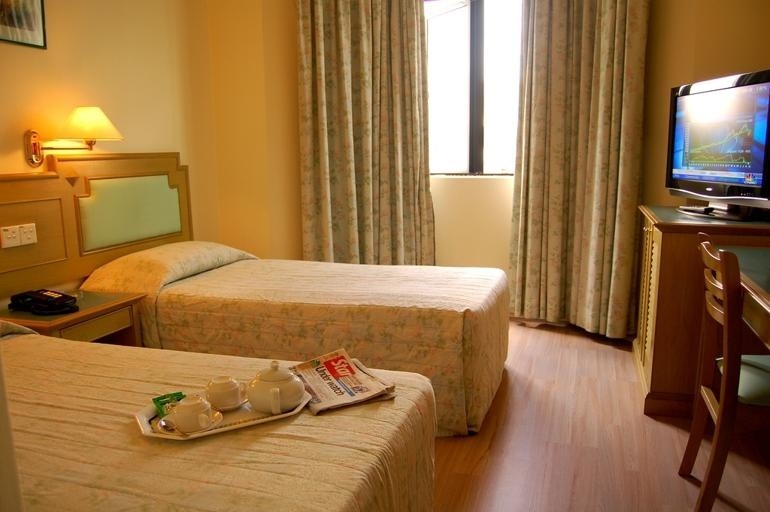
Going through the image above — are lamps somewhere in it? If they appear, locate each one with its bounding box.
[23,107,124,169]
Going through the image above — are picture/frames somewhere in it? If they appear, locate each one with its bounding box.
[0,0,47,49]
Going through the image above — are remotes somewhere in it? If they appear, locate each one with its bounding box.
[679,204,715,215]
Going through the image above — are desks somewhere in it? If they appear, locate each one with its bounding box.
[1,287,148,340]
[716,246,770,349]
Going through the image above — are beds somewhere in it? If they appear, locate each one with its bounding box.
[48,152,510,438]
[0,318,438,511]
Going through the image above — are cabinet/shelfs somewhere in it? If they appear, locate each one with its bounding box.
[630,204,770,415]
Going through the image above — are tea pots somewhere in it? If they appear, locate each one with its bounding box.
[247,360,306,416]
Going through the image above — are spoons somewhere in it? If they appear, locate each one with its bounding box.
[160,418,190,439]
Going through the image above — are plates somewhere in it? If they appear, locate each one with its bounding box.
[158,411,223,437]
[215,398,249,412]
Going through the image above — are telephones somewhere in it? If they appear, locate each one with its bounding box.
[9,289,79,315]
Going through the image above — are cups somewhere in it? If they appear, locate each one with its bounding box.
[206,374,247,406]
[172,394,213,434]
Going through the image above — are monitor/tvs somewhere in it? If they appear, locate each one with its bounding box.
[665,70,770,222]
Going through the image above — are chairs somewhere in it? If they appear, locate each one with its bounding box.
[676,231,770,512]
